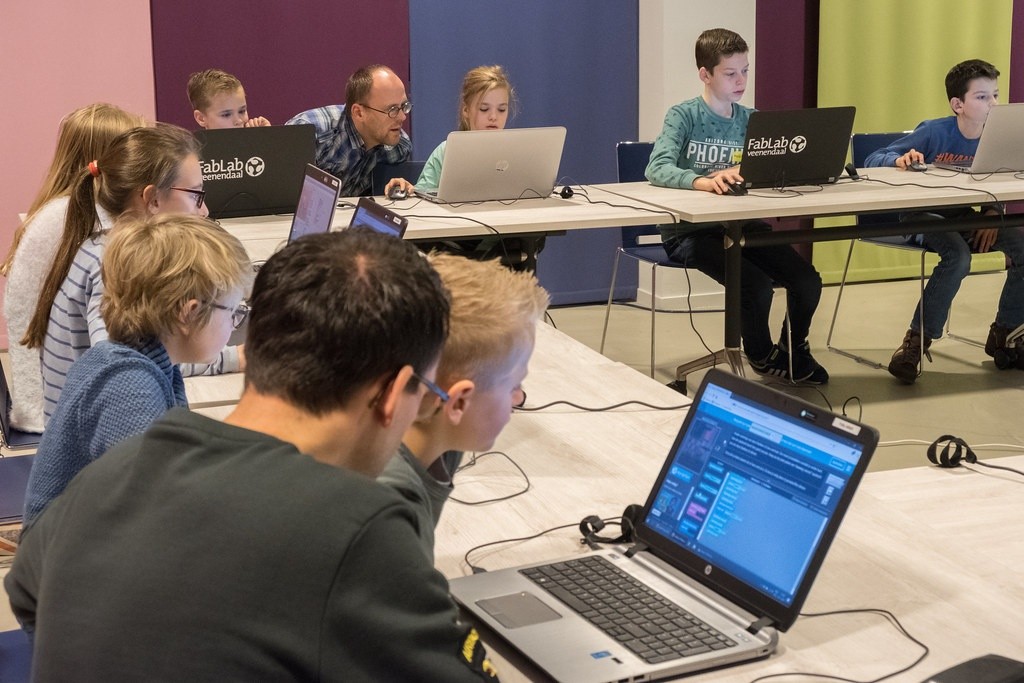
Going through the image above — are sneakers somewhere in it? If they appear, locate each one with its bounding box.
[781,340,829,384]
[889,330,932,382]
[749,344,816,382]
[985,322,1024,371]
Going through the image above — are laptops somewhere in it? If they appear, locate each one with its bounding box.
[413,126,568,204]
[447,369,882,683]
[735,105,857,191]
[932,103,1024,175]
[191,124,409,275]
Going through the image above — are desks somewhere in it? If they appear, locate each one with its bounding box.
[169,164,1024,683]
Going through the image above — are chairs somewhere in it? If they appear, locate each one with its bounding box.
[827,133,986,376]
[0,359,43,451]
[0,453,37,526]
[599,141,698,379]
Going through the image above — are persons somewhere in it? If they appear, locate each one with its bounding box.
[0,102,149,434]
[21,212,255,529]
[284,64,413,198]
[370,250,550,563]
[19,123,209,430]
[384,64,538,275]
[186,69,271,131]
[863,59,1024,384]
[3,223,501,683]
[645,28,829,387]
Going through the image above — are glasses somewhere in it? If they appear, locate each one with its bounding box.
[367,372,451,424]
[358,102,414,118]
[141,187,206,208]
[192,298,248,328]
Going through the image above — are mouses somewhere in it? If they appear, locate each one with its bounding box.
[388,184,409,200]
[905,159,928,172]
[718,182,750,196]
